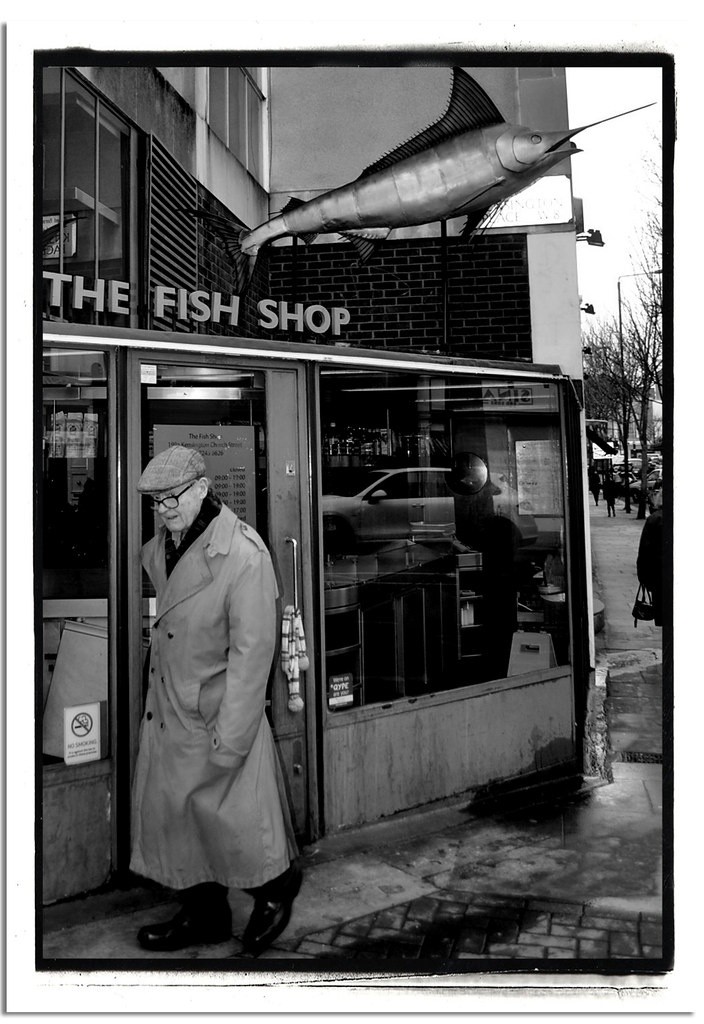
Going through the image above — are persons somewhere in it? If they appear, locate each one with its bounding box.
[602,473,618,517]
[128,445,304,953]
[588,470,601,506]
[636,488,663,628]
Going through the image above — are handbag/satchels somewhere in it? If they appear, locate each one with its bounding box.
[632,584,655,621]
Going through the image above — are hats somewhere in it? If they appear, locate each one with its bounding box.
[136,445,205,495]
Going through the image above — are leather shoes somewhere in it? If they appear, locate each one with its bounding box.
[137,899,233,952]
[242,869,303,952]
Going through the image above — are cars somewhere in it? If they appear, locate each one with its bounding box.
[323,466,457,554]
[630,468,663,502]
